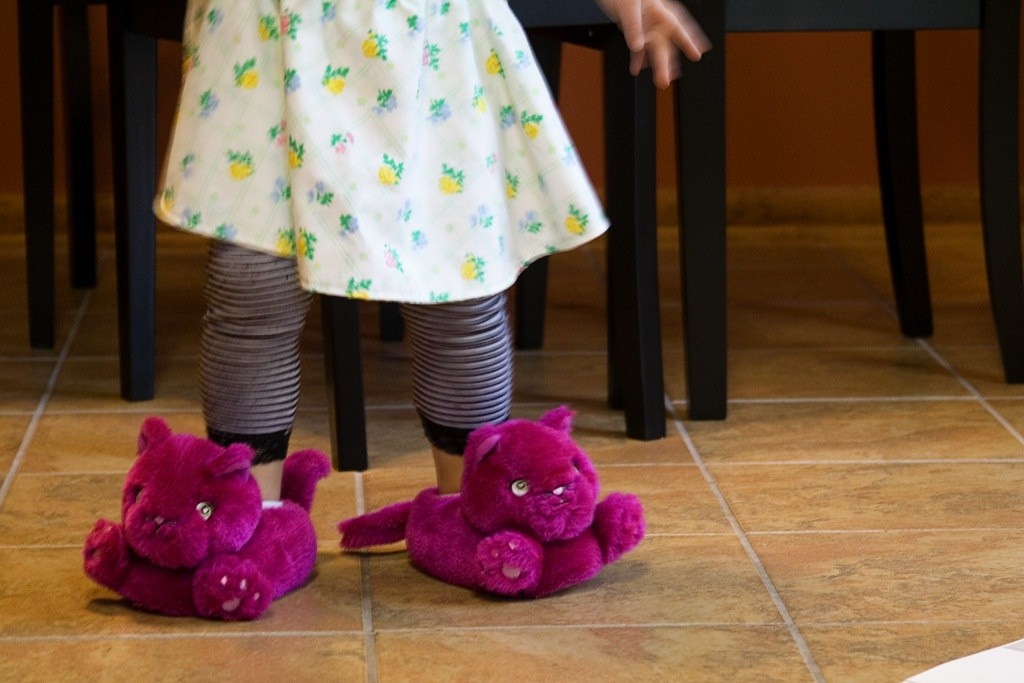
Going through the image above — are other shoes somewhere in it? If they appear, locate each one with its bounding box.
[337,406,641,595]
[84,415,330,621]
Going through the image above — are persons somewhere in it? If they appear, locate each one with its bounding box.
[84,0,704,624]
